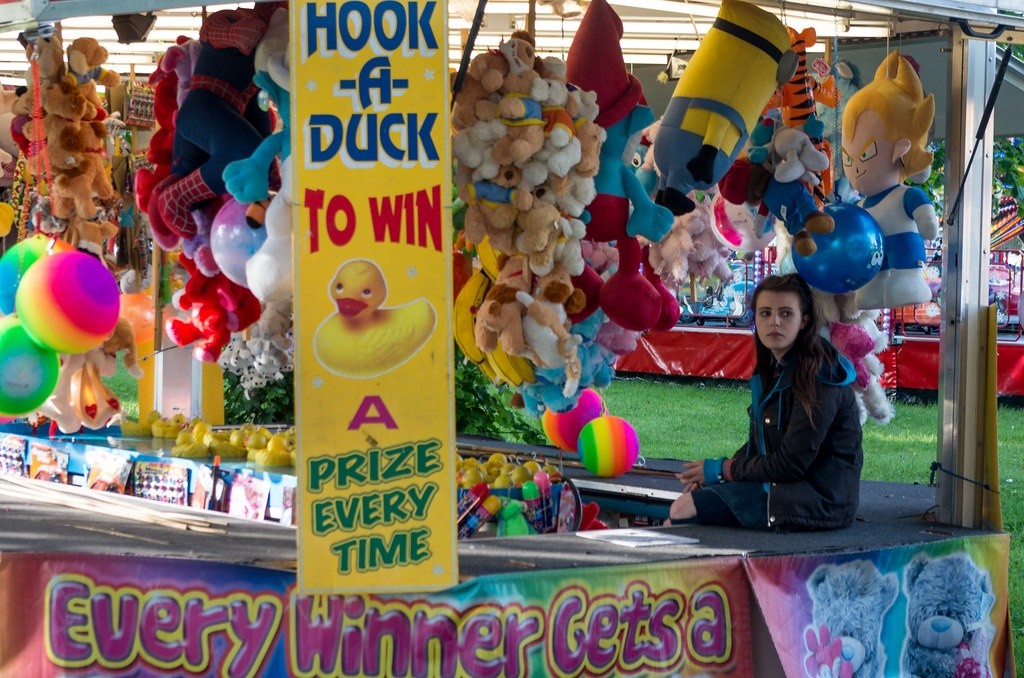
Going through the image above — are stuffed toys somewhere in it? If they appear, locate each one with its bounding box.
[0,0,294,436]
[804,551,997,678]
[449,0,940,424]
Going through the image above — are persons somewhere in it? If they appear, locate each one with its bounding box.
[664,271,864,533]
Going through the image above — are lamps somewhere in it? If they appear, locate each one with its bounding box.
[667,50,697,81]
[112,13,156,44]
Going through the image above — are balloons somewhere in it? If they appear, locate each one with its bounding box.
[211,191,279,287]
[712,193,776,252]
[792,200,886,294]
